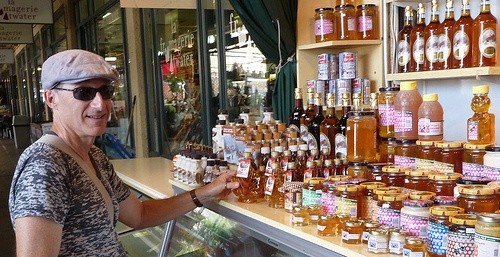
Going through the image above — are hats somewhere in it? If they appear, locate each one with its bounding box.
[40,49,118,90]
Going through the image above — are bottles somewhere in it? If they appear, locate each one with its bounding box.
[172,0,500,209]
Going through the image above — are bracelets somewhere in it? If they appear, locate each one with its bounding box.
[189,189,204,207]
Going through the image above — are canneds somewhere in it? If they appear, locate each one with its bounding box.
[313,0,379,43]
[306,51,371,107]
[279,139,500,257]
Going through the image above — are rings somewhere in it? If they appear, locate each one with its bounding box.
[225,182,228,189]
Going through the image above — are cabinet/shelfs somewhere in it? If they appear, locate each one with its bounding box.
[380,0,500,169]
[296,0,384,104]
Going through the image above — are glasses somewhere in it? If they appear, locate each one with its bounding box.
[53,84,115,101]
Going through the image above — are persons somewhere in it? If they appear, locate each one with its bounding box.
[9,50,240,257]
